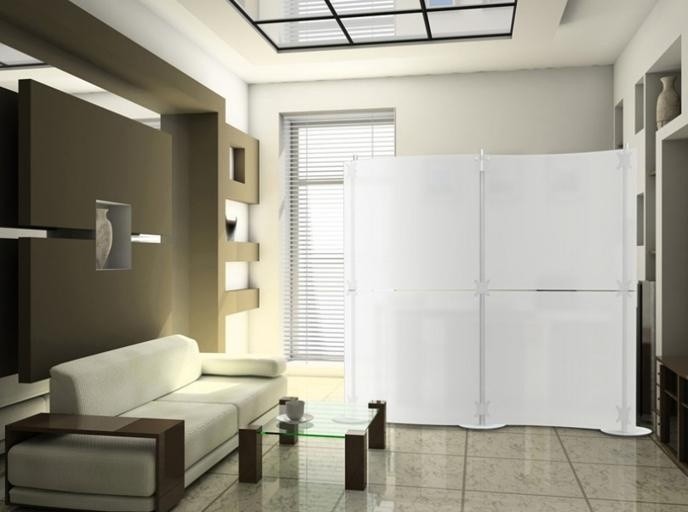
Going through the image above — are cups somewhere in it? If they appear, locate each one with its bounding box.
[285,400,305,421]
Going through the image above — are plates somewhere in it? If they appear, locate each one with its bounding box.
[278,413,312,425]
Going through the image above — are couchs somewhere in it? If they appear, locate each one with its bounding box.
[5,332,291,510]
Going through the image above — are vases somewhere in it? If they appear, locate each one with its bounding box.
[95,207,114,267]
[652,73,679,128]
